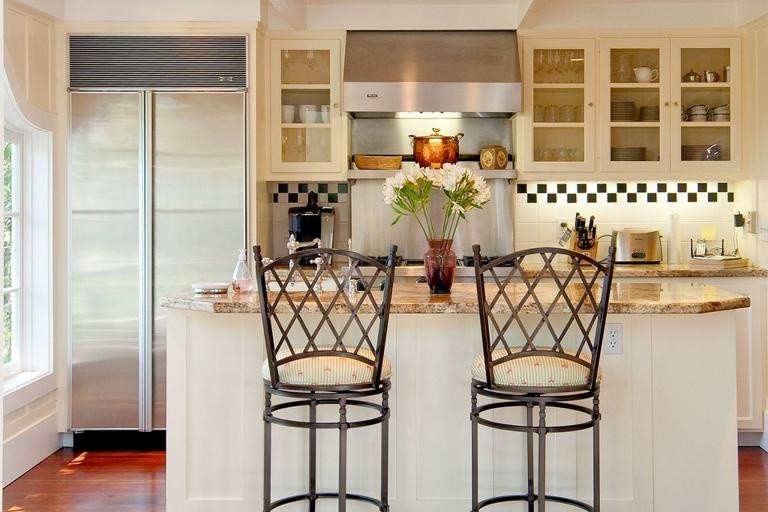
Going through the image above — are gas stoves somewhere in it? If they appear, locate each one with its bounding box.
[342,256,524,283]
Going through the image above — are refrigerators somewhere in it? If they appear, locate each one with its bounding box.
[67,90,246,433]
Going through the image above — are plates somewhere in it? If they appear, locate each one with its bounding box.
[611,101,635,122]
[611,146,648,161]
[682,145,721,161]
[191,284,230,294]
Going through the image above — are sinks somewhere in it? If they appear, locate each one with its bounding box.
[267,276,345,293]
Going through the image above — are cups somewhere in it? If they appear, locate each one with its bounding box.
[705,71,720,83]
[575,105,584,121]
[321,105,330,123]
[282,105,295,122]
[299,105,317,124]
[533,49,584,83]
[561,105,575,122]
[534,104,544,122]
[640,106,658,121]
[546,104,559,123]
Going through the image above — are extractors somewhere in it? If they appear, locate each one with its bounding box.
[343,31,523,113]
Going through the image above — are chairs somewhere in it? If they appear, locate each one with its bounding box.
[469,242,616,512]
[252,243,398,512]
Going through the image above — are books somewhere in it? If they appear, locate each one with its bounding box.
[688,258,749,270]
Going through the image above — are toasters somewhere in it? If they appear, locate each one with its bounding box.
[611,229,664,264]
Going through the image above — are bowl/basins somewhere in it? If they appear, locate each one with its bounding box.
[681,105,687,113]
[354,155,402,170]
[687,104,710,114]
[682,115,689,121]
[711,103,729,114]
[689,115,710,122]
[712,114,729,122]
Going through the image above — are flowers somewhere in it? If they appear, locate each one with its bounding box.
[382,163,492,239]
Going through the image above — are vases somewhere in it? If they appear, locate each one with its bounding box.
[423,239,457,303]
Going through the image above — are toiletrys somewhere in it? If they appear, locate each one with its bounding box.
[231,248,254,294]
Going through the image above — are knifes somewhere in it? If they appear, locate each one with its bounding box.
[568,212,599,267]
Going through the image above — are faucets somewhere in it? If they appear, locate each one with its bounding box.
[261,234,324,294]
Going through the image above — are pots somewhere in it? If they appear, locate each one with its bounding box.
[409,128,464,169]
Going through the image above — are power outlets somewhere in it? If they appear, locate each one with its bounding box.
[734,212,756,234]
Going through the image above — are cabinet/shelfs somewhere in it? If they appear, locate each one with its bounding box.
[349,121,517,179]
[263,29,348,183]
[517,32,598,181]
[598,29,749,182]
[670,281,766,446]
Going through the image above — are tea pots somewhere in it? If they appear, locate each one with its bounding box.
[634,66,659,83]
